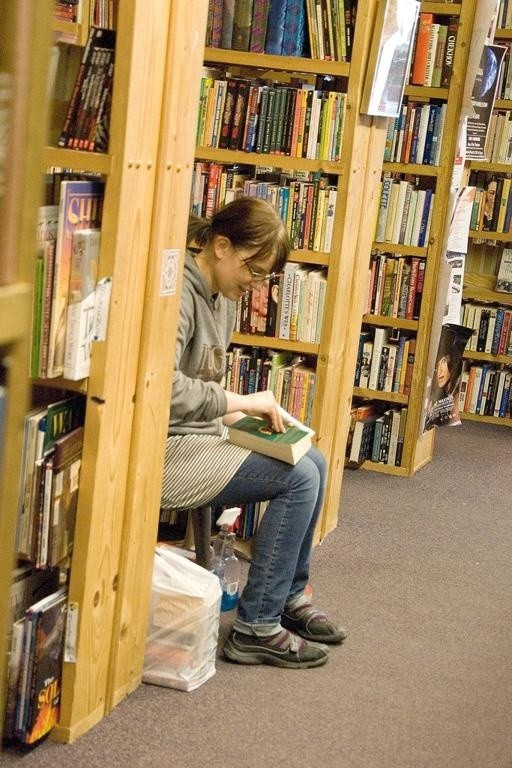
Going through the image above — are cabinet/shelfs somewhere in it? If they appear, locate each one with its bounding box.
[458,0,512,430]
[346,0,495,480]
[189,1,418,550]
[2,3,211,753]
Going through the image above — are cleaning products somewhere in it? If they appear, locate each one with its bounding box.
[210,506,243,611]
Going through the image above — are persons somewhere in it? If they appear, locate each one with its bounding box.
[435,346,456,396]
[151,201,345,669]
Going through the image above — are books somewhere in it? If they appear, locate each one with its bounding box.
[373,9,461,248]
[152,1,360,550]
[339,255,429,470]
[455,299,510,421]
[1,0,119,756]
[466,0,511,165]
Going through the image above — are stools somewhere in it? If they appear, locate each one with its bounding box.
[157,493,258,573]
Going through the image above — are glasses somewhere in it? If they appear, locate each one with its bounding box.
[231,243,283,285]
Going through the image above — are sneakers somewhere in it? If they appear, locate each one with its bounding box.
[283,599,350,642]
[224,623,328,670]
